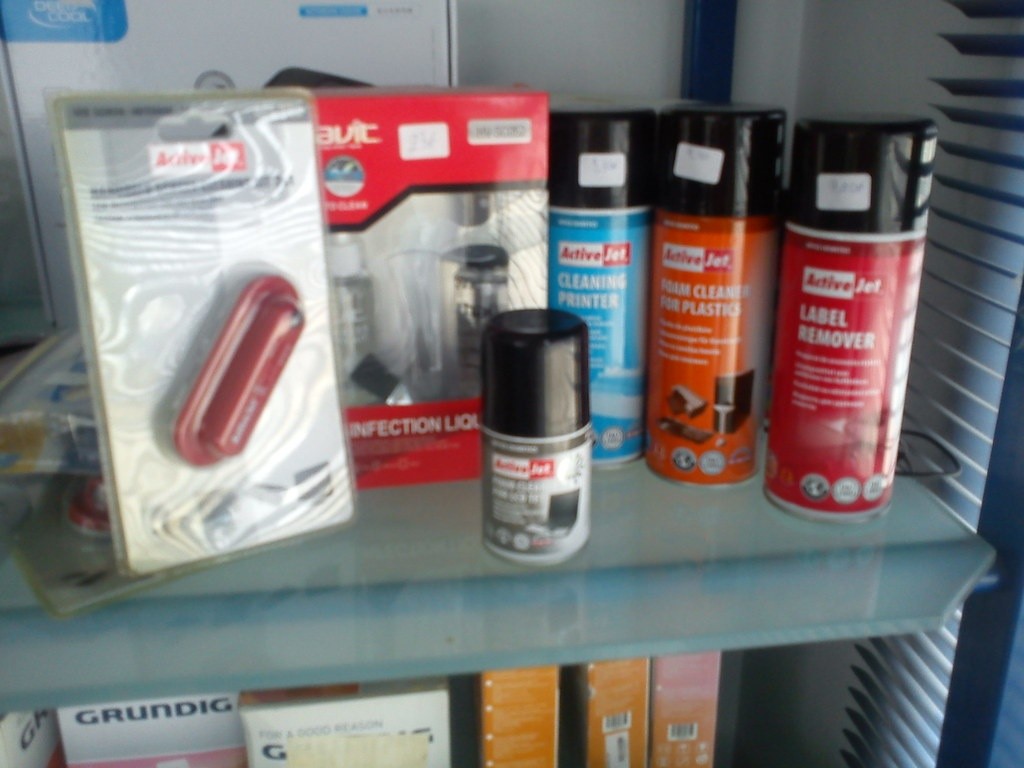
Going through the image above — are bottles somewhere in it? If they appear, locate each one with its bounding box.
[480,312,593,563]
[645,103,785,487]
[553,108,652,466]
[763,113,940,520]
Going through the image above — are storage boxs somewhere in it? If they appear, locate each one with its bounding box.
[313,83,552,493]
[0,646,725,768]
